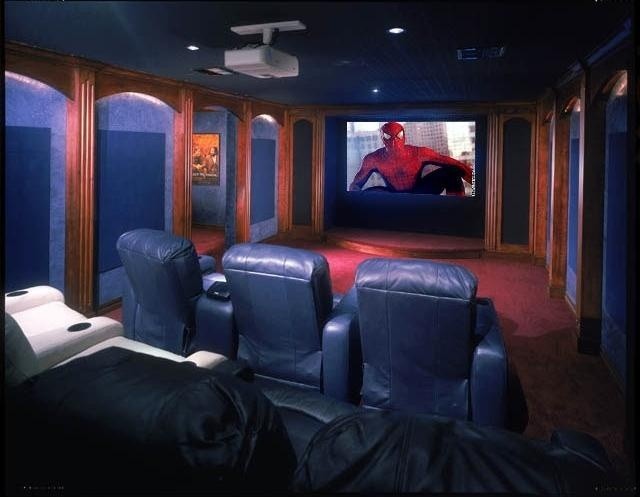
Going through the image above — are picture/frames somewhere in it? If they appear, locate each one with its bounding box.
[192,131,220,186]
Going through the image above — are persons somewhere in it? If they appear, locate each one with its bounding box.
[204,145,219,174]
[350,121,473,196]
[192,145,208,175]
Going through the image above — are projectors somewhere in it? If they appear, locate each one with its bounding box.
[223,44,299,78]
